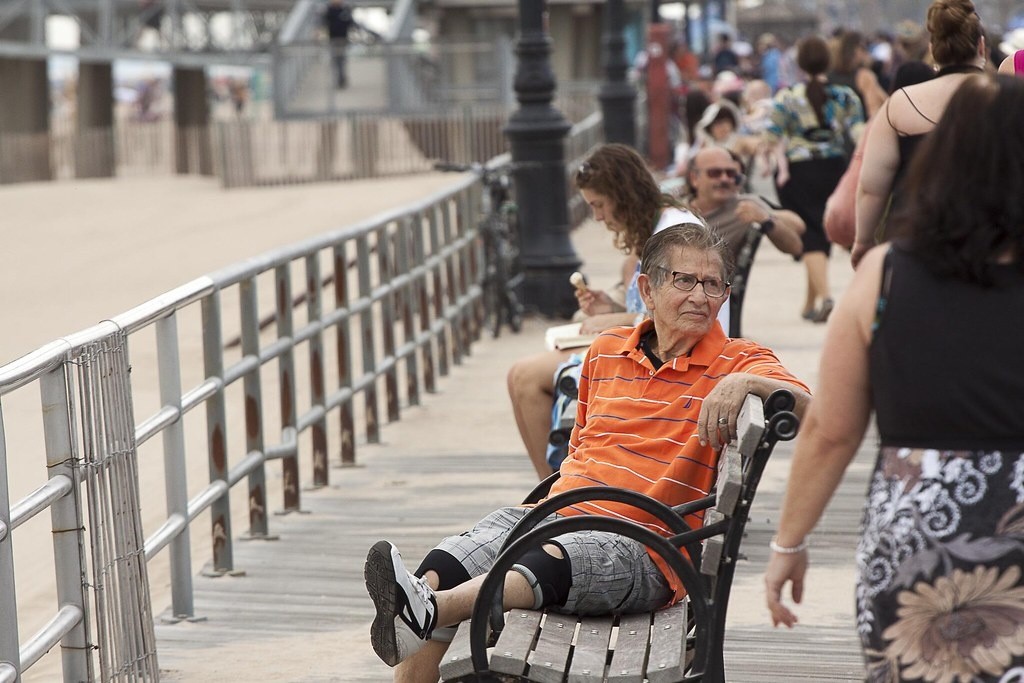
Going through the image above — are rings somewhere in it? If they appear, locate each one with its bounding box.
[719,418,729,424]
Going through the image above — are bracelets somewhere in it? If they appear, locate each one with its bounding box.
[770,534,810,553]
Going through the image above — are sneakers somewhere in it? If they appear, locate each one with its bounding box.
[365,540,439,667]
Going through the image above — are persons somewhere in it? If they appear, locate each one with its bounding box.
[506,143,712,483]
[218,76,251,118]
[321,2,357,88]
[131,78,158,117]
[367,224,811,683]
[620,1,1024,683]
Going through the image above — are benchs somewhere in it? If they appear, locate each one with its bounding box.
[437,388,799,683]
[547,216,775,446]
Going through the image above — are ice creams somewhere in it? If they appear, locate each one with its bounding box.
[569,272,587,292]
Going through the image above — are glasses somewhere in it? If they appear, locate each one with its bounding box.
[658,266,731,299]
[696,167,738,178]
[579,162,601,176]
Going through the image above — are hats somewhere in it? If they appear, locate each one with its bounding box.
[742,80,773,103]
[714,70,743,93]
[998,28,1024,55]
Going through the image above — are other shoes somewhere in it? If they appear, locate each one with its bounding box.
[803,298,834,322]
